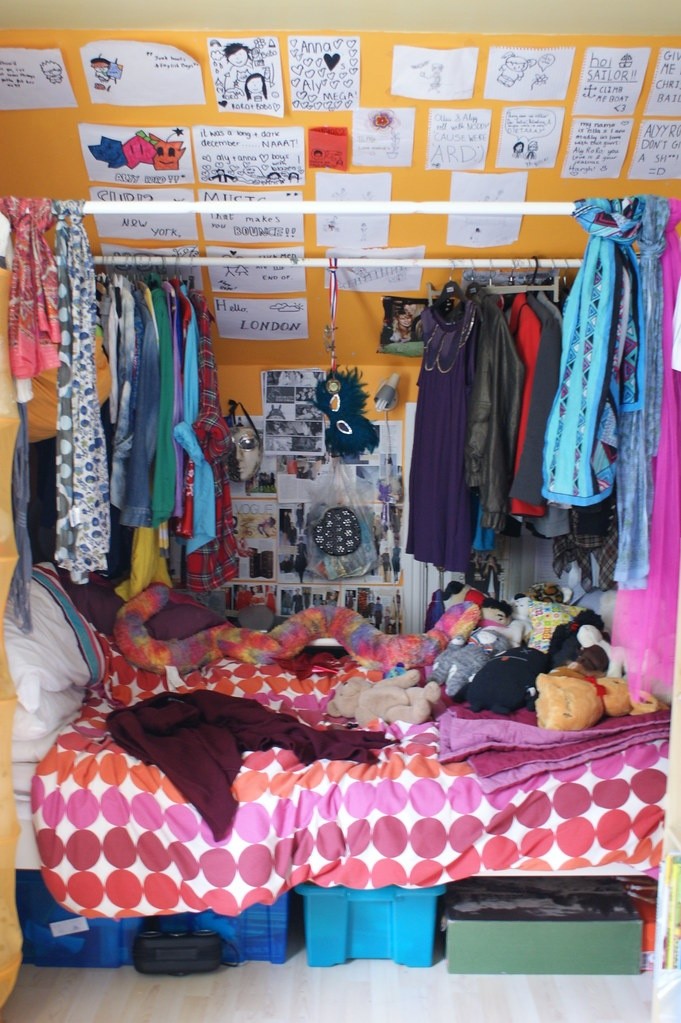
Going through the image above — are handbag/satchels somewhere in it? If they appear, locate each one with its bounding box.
[304,458,380,580]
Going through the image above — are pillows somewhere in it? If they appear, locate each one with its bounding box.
[2,562,106,715]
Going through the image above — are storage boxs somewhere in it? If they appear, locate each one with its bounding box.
[15,869,290,969]
[294,881,448,970]
[443,898,644,975]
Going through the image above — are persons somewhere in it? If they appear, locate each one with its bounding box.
[382,301,426,345]
[236,370,402,635]
[468,554,500,602]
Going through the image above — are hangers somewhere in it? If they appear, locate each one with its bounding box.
[433,257,572,305]
[95,249,200,286]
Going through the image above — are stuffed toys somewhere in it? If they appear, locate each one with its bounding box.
[326,669,441,727]
[113,585,480,675]
[426,582,669,731]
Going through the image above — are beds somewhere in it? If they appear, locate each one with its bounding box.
[10,641,671,920]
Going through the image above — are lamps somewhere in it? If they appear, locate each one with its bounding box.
[375,373,400,413]
